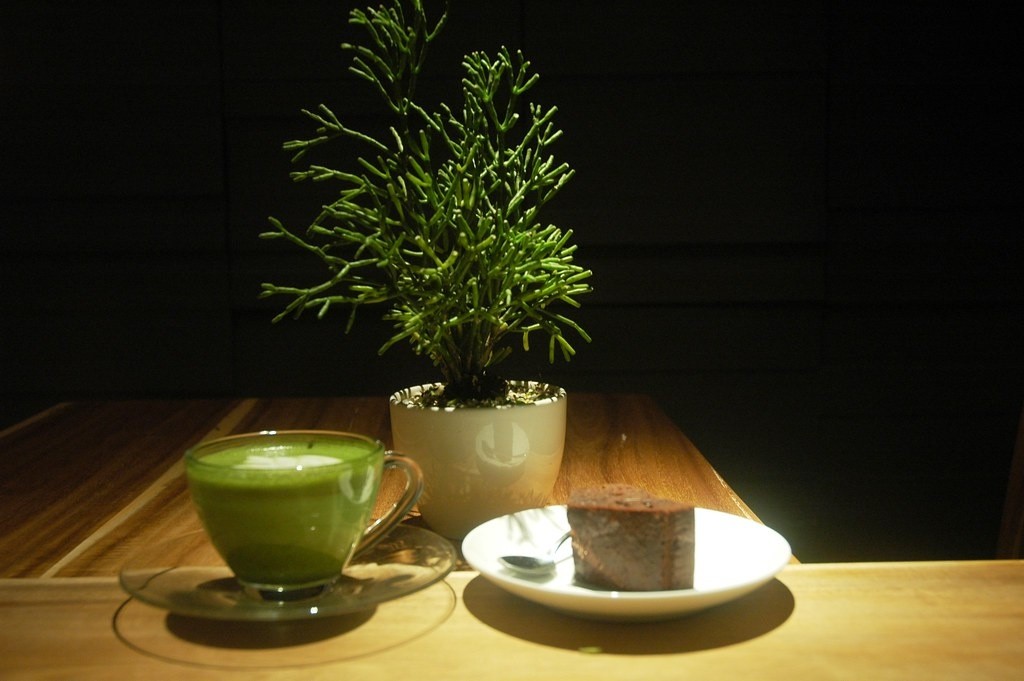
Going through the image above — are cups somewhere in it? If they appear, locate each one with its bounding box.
[182,429,423,594]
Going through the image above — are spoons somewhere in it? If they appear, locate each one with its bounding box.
[497,531,573,575]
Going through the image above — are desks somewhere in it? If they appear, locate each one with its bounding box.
[0,394,1024,681]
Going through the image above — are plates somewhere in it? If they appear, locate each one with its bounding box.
[118,520,457,621]
[461,505,790,622]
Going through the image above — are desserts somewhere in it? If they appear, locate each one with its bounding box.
[568,486,696,593]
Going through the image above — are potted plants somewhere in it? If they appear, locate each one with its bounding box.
[256,0,595,541]
